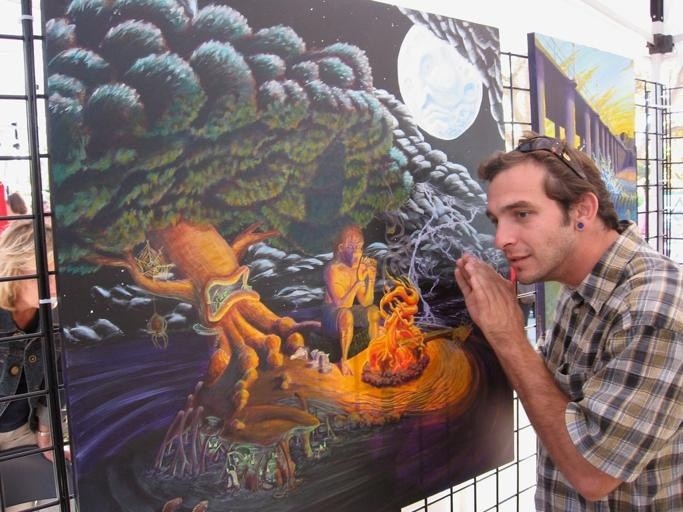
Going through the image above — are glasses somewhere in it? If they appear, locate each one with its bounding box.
[507,135,590,183]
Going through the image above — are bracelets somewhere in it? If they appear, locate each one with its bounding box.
[33,429,50,438]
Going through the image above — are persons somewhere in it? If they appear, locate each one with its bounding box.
[318,224,380,379]
[5,192,27,219]
[0,212,76,511]
[452,129,683,512]
